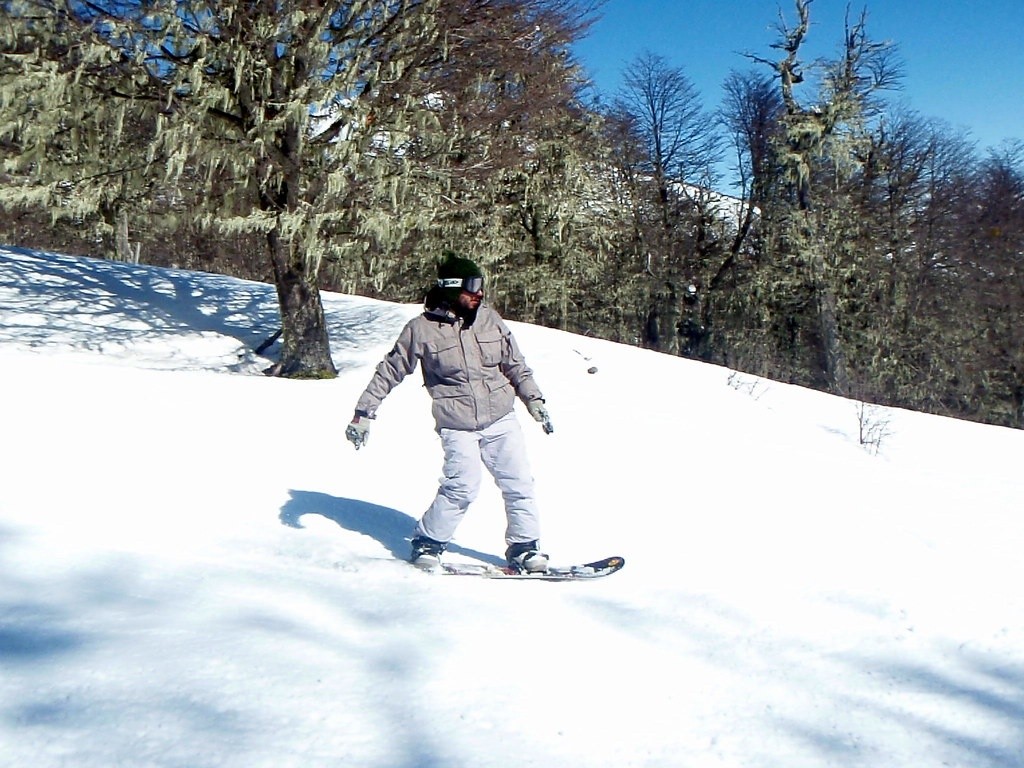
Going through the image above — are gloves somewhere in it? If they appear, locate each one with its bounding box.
[528,400,554,435]
[345,416,371,450]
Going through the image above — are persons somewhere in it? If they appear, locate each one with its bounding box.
[345,256,553,571]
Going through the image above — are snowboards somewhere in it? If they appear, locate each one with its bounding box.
[365,555,628,582]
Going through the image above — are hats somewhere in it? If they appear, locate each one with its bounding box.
[437,257,486,310]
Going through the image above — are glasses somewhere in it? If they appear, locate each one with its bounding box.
[436,276,486,294]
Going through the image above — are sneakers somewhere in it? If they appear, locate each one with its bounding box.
[410,535,441,567]
[505,550,547,572]
